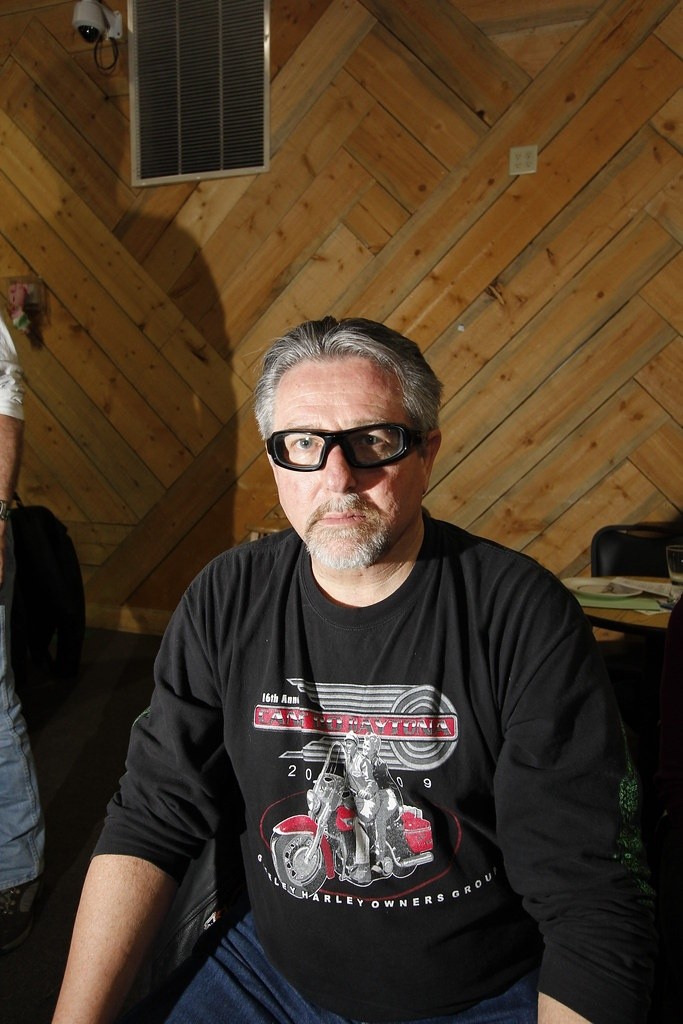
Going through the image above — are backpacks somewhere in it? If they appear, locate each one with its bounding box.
[12,491,85,691]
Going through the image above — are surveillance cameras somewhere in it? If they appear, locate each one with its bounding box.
[71,2,105,43]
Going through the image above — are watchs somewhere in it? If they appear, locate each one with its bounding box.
[0,501,10,522]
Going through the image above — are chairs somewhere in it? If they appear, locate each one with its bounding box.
[589,524,683,578]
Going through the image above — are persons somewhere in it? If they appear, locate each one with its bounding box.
[0,319,46,955]
[50,316,663,1024]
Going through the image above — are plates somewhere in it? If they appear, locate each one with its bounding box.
[561,577,643,598]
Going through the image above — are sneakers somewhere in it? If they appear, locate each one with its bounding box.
[0,861,45,950]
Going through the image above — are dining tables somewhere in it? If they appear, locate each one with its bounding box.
[560,576,682,635]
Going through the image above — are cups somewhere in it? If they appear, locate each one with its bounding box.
[666,546,683,606]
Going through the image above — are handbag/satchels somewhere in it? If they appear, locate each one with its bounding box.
[151,830,230,976]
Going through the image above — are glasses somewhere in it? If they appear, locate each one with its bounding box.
[267,422,423,473]
